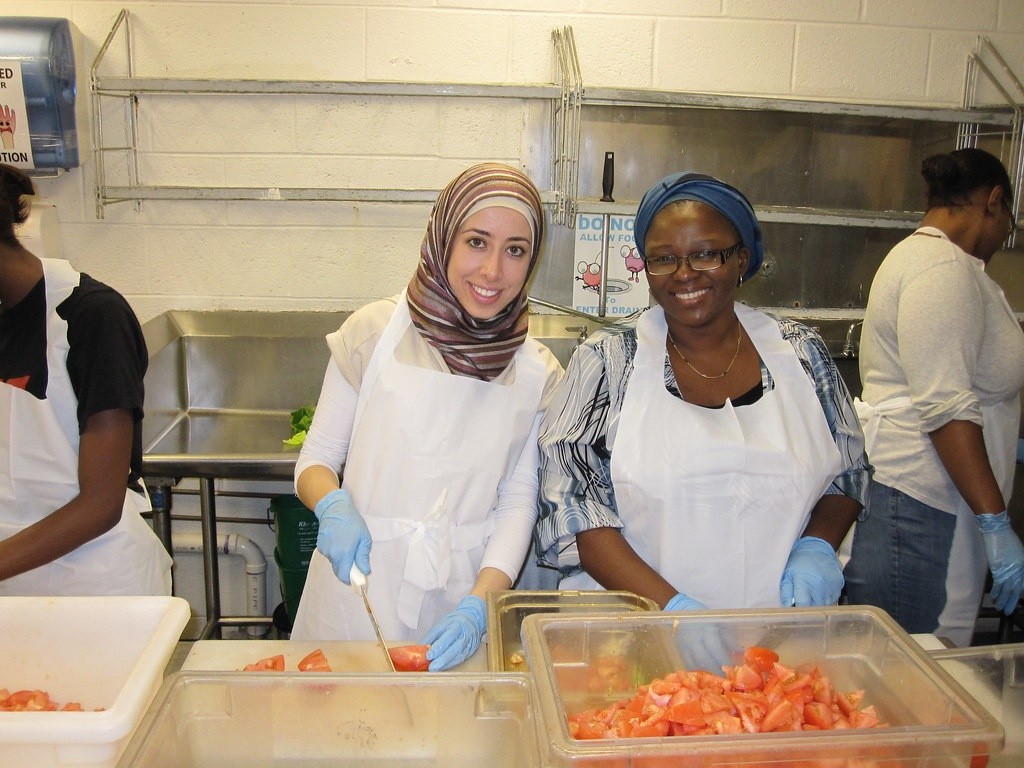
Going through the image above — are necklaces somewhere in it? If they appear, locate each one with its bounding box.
[667,322,741,379]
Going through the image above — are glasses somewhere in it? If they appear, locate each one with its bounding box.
[642,240,742,276]
[1005,200,1015,235]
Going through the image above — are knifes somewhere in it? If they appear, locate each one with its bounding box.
[350,559,414,728]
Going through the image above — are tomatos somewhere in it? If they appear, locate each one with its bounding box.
[297,649,332,672]
[240,654,285,672]
[387,645,431,672]
[0,688,105,712]
[566,645,907,768]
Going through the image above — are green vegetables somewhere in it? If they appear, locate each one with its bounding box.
[282,406,314,445]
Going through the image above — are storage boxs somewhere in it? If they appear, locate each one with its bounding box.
[0,587,1009,768]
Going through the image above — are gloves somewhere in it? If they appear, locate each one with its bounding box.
[664,593,744,680]
[315,490,372,586]
[781,536,844,608]
[975,508,1024,614]
[418,594,488,672]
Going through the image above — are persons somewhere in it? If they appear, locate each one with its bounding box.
[288,162,565,671]
[0,164,173,597]
[846,148,1024,634]
[534,171,876,678]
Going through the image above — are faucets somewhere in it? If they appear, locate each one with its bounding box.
[565,326,589,345]
